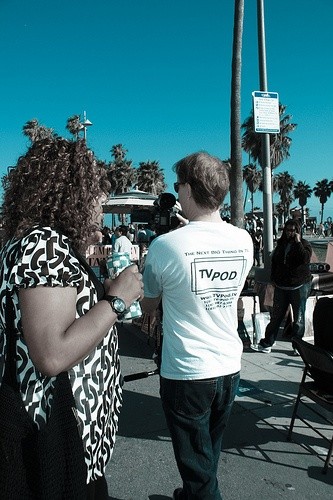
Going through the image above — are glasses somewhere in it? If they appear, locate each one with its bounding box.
[174,182,187,193]
[284,228,297,233]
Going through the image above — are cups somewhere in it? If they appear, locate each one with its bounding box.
[105,252,143,322]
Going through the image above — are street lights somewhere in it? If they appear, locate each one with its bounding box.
[80,110,93,144]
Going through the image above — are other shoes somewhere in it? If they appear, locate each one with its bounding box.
[250,343,272,353]
[173,487,185,499]
[293,348,300,356]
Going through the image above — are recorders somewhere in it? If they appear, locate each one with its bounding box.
[309,262,330,272]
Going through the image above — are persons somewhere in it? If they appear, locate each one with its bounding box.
[222,214,333,266]
[249,218,314,354]
[0,136,145,500]
[91,218,157,268]
[142,152,254,500]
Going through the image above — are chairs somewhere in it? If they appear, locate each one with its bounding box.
[287,337,333,476]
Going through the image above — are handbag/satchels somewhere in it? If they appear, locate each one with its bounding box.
[0,287,89,499]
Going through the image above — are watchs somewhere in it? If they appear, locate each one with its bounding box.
[99,294,128,321]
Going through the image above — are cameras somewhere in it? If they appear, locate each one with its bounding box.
[131,193,185,230]
